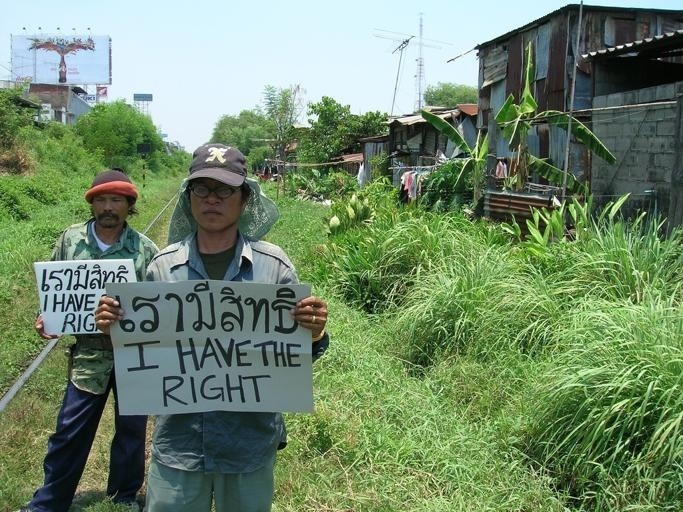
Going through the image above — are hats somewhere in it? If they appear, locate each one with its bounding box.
[187,143,248,185]
[84,170,138,202]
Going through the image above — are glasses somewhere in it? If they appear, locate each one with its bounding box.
[187,186,242,200]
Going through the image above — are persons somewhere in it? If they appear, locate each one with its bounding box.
[92,142,330,511]
[16,170,160,511]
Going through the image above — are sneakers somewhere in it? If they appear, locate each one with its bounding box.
[116,501,141,512]
[16,502,32,512]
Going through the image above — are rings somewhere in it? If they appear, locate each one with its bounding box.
[311,315,315,324]
